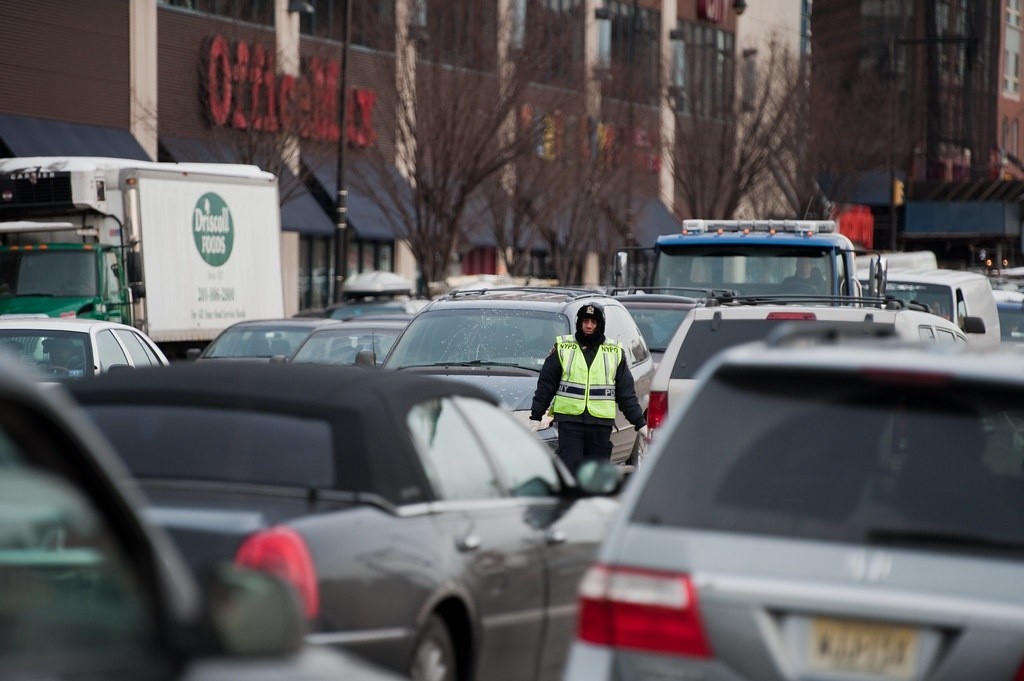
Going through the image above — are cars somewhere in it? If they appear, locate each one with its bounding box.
[43,362,621,681]
[189,318,407,379]
[0,315,169,393]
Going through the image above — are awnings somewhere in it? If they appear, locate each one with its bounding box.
[0,112,152,163]
[422,170,683,256]
[159,134,417,238]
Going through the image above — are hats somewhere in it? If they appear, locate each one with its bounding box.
[575,303,606,346]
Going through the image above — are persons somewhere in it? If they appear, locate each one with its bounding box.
[529,302,647,475]
[38,338,85,376]
[539,318,562,344]
[776,257,828,294]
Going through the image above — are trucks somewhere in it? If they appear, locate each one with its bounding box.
[611,217,888,307]
[0,157,283,396]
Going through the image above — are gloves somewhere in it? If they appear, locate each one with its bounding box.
[528,420,540,432]
[638,424,648,439]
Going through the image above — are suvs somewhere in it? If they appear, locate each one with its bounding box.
[374,288,749,491]
[638,294,970,453]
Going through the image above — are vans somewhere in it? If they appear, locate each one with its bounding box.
[859,269,1003,348]
[565,337,1024,681]
